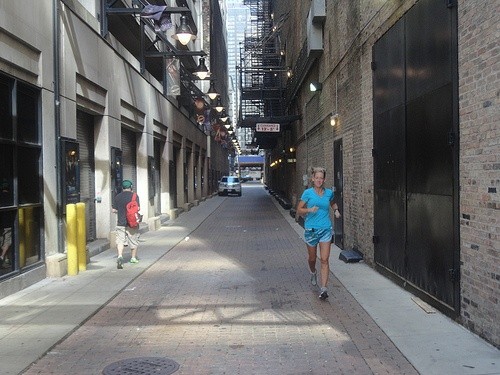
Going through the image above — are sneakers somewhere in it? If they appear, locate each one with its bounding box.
[319,290,329,299]
[311,271,317,286]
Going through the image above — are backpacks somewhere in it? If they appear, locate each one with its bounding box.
[126,193,140,227]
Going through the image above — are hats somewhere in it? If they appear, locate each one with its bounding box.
[122,180,131,189]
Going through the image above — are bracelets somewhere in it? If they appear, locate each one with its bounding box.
[334,209,338,212]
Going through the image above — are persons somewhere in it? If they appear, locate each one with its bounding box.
[297,167,340,299]
[66,155,76,185]
[0,186,13,265]
[112,180,140,269]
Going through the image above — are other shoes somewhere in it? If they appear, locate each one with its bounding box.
[130,259,141,263]
[117,256,123,269]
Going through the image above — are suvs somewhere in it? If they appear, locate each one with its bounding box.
[217,176,243,197]
